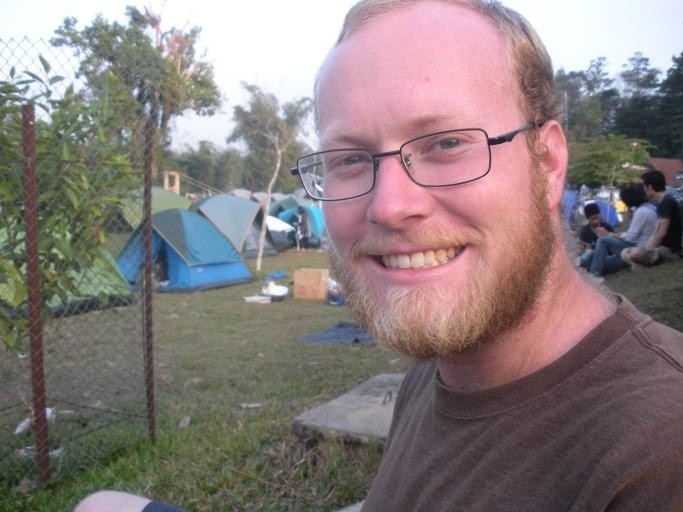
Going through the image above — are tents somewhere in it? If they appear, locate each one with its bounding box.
[562,189,621,228]
[228,189,324,249]
[186,193,279,258]
[112,207,256,293]
[97,189,192,235]
[1,226,134,321]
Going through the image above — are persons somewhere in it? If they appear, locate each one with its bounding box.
[573,204,616,272]
[579,186,657,277]
[69,1,683,512]
[619,170,683,273]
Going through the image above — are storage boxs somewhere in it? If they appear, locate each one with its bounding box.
[292,268,329,302]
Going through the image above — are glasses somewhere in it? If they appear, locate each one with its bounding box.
[291,117,544,201]
